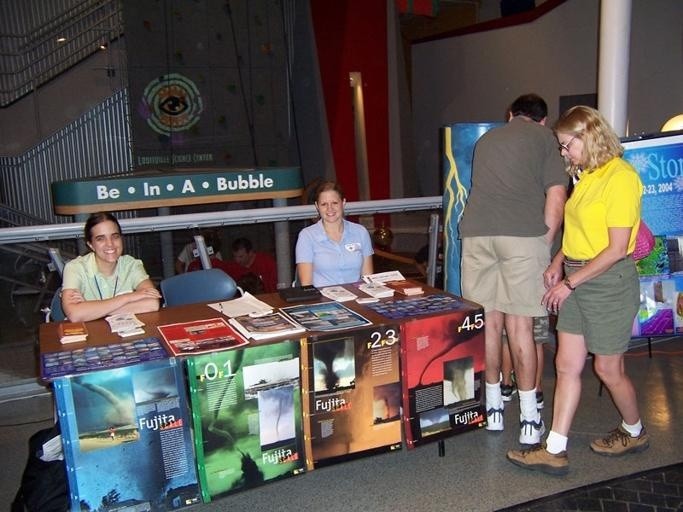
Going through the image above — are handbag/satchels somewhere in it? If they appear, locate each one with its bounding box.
[631,219,655,260]
[11,425,70,512]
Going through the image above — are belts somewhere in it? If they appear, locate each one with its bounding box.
[564,258,589,268]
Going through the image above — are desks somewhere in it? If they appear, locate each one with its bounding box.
[36,271,485,511]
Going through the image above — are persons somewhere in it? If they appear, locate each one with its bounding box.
[292,178,375,286]
[109,426,115,440]
[458,95,566,447]
[507,106,649,477]
[177,228,228,272]
[224,235,276,291]
[61,212,163,324]
[415,222,445,284]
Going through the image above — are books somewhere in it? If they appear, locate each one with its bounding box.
[319,286,358,303]
[279,284,322,303]
[357,283,395,300]
[384,280,423,296]
[56,321,87,344]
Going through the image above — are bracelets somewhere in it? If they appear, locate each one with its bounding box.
[562,279,576,294]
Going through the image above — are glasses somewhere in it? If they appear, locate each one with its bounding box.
[558,136,575,153]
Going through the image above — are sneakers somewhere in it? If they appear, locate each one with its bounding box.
[507,443,568,476]
[518,413,545,444]
[536,392,543,410]
[500,381,516,401]
[485,403,503,432]
[587,423,649,457]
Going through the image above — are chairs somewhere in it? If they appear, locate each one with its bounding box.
[291,266,303,290]
[11,244,57,318]
[155,270,243,309]
[41,285,71,325]
